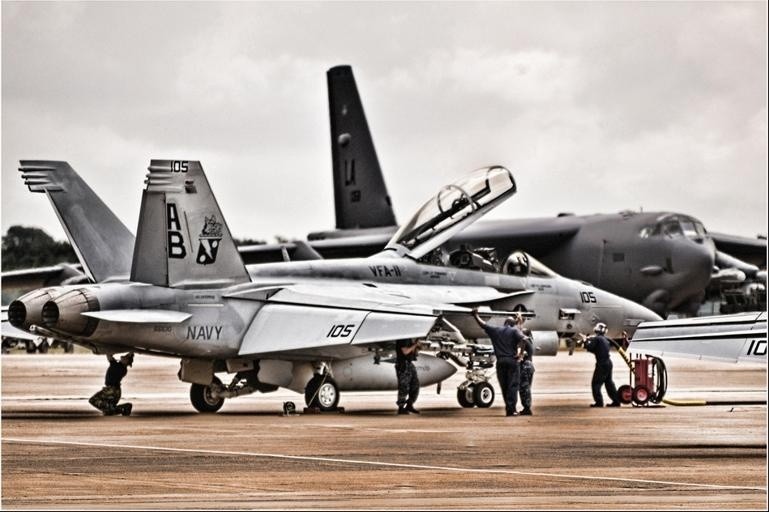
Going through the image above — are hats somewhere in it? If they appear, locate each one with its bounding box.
[120,354,133,368]
[592,322,608,334]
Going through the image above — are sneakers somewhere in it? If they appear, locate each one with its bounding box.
[590,401,604,407]
[397,405,420,415]
[606,401,621,407]
[122,402,132,416]
[505,406,533,416]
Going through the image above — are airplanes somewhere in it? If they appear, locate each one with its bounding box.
[236,63,768,322]
[2,155,766,413]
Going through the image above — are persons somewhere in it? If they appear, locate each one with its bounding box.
[89,342,134,416]
[393,339,420,415]
[578,322,621,408]
[470,308,534,416]
